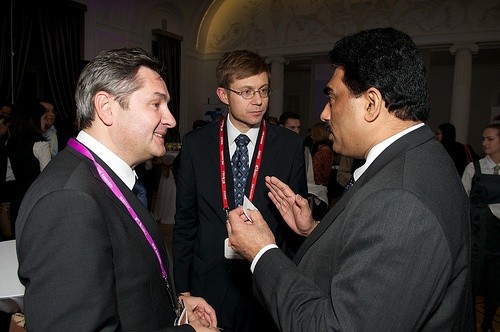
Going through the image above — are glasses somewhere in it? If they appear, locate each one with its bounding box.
[219,86,273,99]
[436,132,442,135]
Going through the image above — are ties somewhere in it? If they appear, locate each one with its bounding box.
[494,165,500,175]
[133,180,147,210]
[343,177,355,193]
[231,135,251,208]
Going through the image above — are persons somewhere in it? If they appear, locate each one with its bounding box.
[172,48,309,332]
[436,122,469,179]
[265,111,366,220]
[461,123,500,332]
[0,100,80,242]
[226,27,473,332]
[14,46,220,332]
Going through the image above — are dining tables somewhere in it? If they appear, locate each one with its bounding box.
[153,167,179,225]
[0,240,27,313]
